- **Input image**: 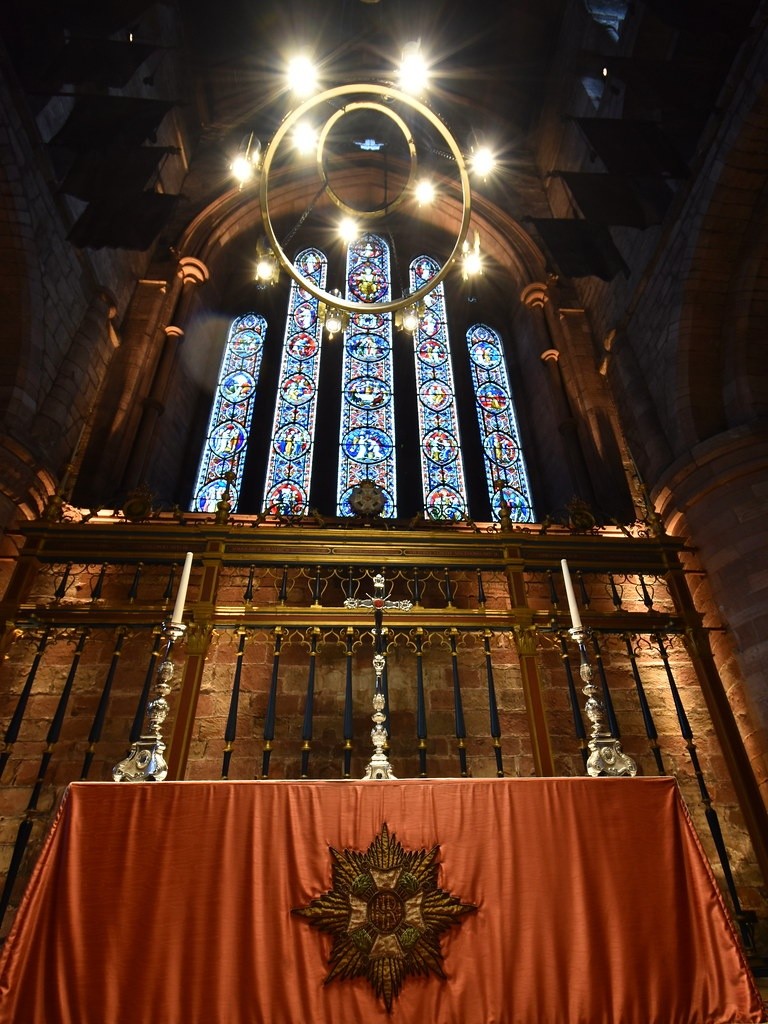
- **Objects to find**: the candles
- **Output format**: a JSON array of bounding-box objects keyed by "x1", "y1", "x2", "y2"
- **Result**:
[
  {"x1": 171, "y1": 552, "x2": 193, "y2": 623},
  {"x1": 561, "y1": 559, "x2": 582, "y2": 628}
]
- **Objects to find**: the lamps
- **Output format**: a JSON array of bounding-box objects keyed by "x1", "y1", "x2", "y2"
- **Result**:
[{"x1": 229, "y1": 78, "x2": 509, "y2": 341}]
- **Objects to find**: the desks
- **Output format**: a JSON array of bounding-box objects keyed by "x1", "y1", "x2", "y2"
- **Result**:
[{"x1": 68, "y1": 776, "x2": 678, "y2": 1024}]
